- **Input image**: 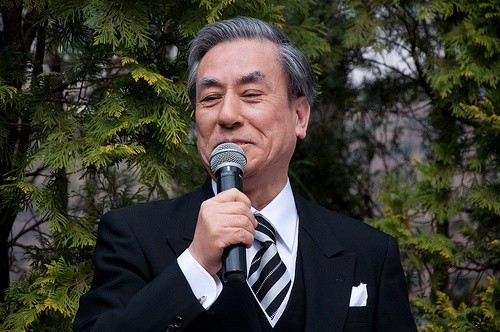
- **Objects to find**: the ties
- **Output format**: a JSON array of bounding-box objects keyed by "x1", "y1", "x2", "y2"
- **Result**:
[{"x1": 246, "y1": 213, "x2": 292, "y2": 320}]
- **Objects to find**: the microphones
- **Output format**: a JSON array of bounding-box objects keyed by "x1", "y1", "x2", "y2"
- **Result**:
[{"x1": 208, "y1": 141, "x2": 247, "y2": 284}]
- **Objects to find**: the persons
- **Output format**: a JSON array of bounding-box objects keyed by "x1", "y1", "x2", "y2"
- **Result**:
[{"x1": 72, "y1": 16, "x2": 414, "y2": 332}]
